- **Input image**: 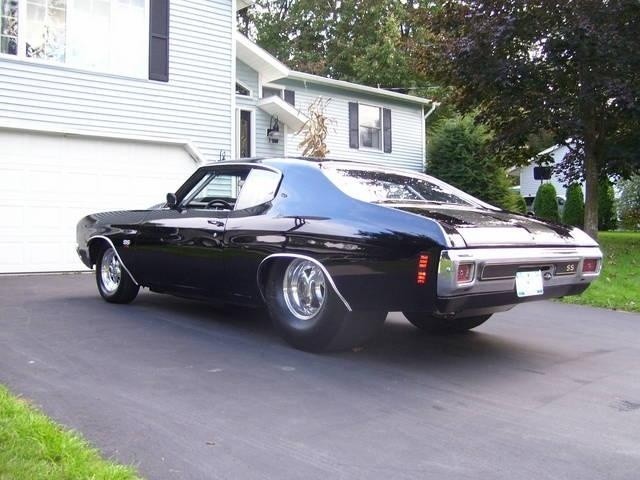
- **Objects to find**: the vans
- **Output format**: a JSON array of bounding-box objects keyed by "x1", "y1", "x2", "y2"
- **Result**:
[{"x1": 520, "y1": 193, "x2": 568, "y2": 220}]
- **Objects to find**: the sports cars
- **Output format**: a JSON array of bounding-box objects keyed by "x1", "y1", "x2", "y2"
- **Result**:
[{"x1": 76, "y1": 155, "x2": 604, "y2": 351}]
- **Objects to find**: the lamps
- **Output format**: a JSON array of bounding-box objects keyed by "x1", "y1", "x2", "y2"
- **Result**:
[{"x1": 267, "y1": 113, "x2": 283, "y2": 143}]
